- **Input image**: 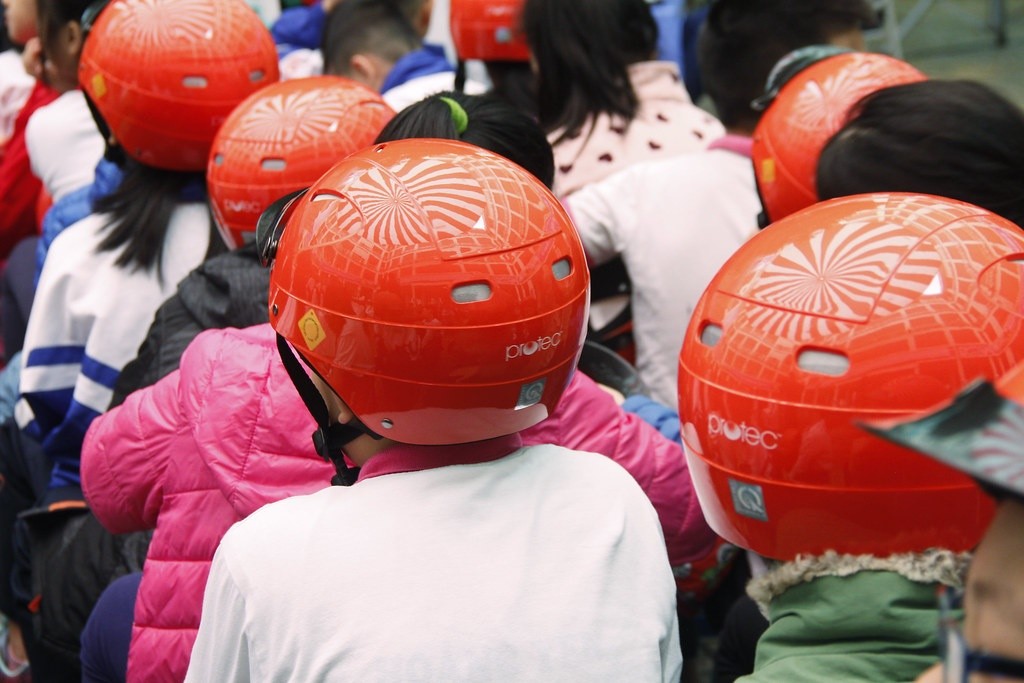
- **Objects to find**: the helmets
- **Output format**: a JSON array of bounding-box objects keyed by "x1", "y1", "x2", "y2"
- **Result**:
[
  {"x1": 268, "y1": 136, "x2": 591, "y2": 446},
  {"x1": 77, "y1": 0, "x2": 280, "y2": 170},
  {"x1": 450, "y1": 0, "x2": 532, "y2": 62},
  {"x1": 860, "y1": 359, "x2": 1024, "y2": 489},
  {"x1": 677, "y1": 190, "x2": 1024, "y2": 562},
  {"x1": 206, "y1": 76, "x2": 398, "y2": 252},
  {"x1": 751, "y1": 50, "x2": 929, "y2": 226}
]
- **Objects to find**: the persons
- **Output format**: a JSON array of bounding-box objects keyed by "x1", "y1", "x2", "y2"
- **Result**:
[
  {"x1": 678, "y1": 196, "x2": 1019, "y2": 683},
  {"x1": 1, "y1": 2, "x2": 1022, "y2": 680},
  {"x1": 176, "y1": 140, "x2": 688, "y2": 683}
]
